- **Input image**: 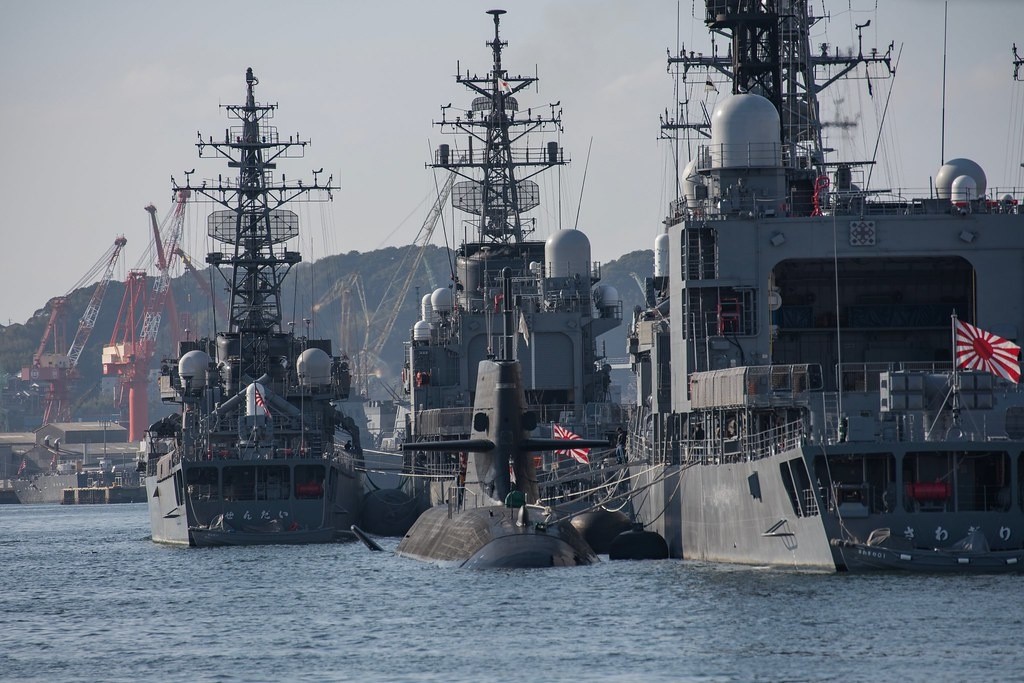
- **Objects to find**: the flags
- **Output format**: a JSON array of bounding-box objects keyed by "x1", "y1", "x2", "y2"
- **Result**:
[
  {"x1": 17, "y1": 459, "x2": 27, "y2": 475},
  {"x1": 508, "y1": 464, "x2": 516, "y2": 484},
  {"x1": 553, "y1": 423, "x2": 591, "y2": 464},
  {"x1": 498, "y1": 77, "x2": 513, "y2": 92},
  {"x1": 256, "y1": 386, "x2": 272, "y2": 419},
  {"x1": 954, "y1": 319, "x2": 1021, "y2": 385},
  {"x1": 518, "y1": 313, "x2": 529, "y2": 347}
]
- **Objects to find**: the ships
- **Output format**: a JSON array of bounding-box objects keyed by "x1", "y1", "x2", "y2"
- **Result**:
[
  {"x1": 142, "y1": 64, "x2": 367, "y2": 545},
  {"x1": 396, "y1": 9, "x2": 633, "y2": 523},
  {"x1": 623, "y1": 1, "x2": 1022, "y2": 572},
  {"x1": 9, "y1": 459, "x2": 148, "y2": 506}
]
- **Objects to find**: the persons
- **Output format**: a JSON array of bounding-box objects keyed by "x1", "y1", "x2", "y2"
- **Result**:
[
  {"x1": 562, "y1": 273, "x2": 584, "y2": 308},
  {"x1": 688, "y1": 421, "x2": 724, "y2": 464},
  {"x1": 839, "y1": 411, "x2": 849, "y2": 443},
  {"x1": 615, "y1": 427, "x2": 627, "y2": 464},
  {"x1": 344, "y1": 439, "x2": 353, "y2": 451},
  {"x1": 731, "y1": 177, "x2": 749, "y2": 200}
]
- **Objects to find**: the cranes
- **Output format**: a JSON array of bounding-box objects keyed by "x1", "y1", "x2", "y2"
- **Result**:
[
  {"x1": 308, "y1": 155, "x2": 464, "y2": 395},
  {"x1": 17, "y1": 187, "x2": 232, "y2": 444}
]
